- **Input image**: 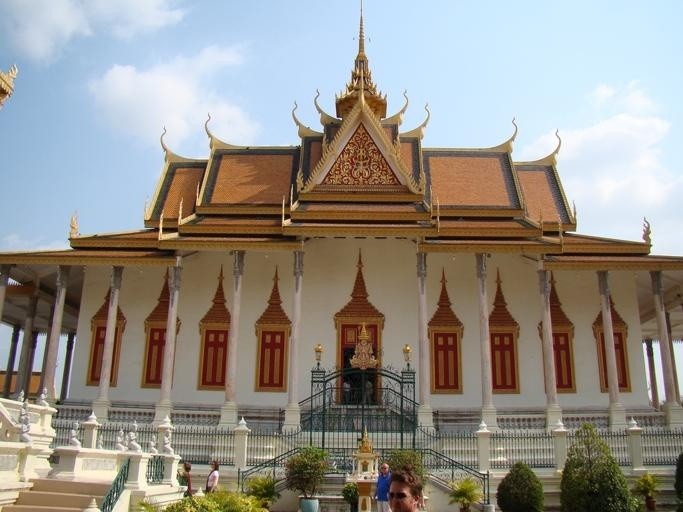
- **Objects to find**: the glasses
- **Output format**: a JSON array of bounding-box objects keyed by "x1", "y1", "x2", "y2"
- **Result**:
[{"x1": 387, "y1": 491, "x2": 415, "y2": 500}]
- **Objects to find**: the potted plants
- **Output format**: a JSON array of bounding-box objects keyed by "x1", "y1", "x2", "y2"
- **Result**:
[
  {"x1": 444, "y1": 474, "x2": 487, "y2": 512},
  {"x1": 283, "y1": 445, "x2": 340, "y2": 512},
  {"x1": 631, "y1": 470, "x2": 660, "y2": 512}
]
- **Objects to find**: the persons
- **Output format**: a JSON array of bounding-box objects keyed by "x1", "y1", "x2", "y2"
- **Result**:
[
  {"x1": 162, "y1": 429, "x2": 174, "y2": 455},
  {"x1": 343, "y1": 379, "x2": 350, "y2": 404},
  {"x1": 371, "y1": 463, "x2": 392, "y2": 512},
  {"x1": 387, "y1": 464, "x2": 422, "y2": 511},
  {"x1": 365, "y1": 379, "x2": 372, "y2": 405},
  {"x1": 19, "y1": 398, "x2": 29, "y2": 422},
  {"x1": 39, "y1": 387, "x2": 49, "y2": 406},
  {"x1": 206, "y1": 461, "x2": 218, "y2": 494},
  {"x1": 16, "y1": 415, "x2": 33, "y2": 442},
  {"x1": 178, "y1": 463, "x2": 192, "y2": 498},
  {"x1": 96, "y1": 435, "x2": 103, "y2": 448},
  {"x1": 126, "y1": 419, "x2": 141, "y2": 450},
  {"x1": 148, "y1": 435, "x2": 158, "y2": 454},
  {"x1": 16, "y1": 389, "x2": 24, "y2": 403},
  {"x1": 69, "y1": 423, "x2": 81, "y2": 447},
  {"x1": 115, "y1": 429, "x2": 126, "y2": 451}
]
